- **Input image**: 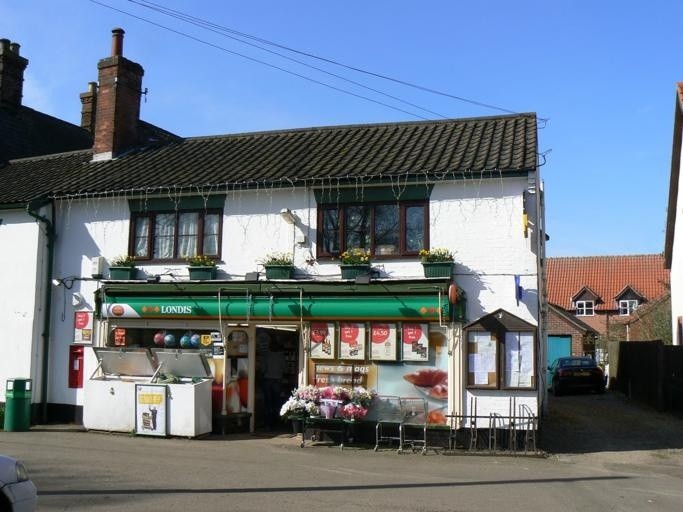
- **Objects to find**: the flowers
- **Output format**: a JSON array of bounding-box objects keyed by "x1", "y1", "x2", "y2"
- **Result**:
[
  {"x1": 263, "y1": 252, "x2": 294, "y2": 265},
  {"x1": 111, "y1": 255, "x2": 136, "y2": 267},
  {"x1": 417, "y1": 248, "x2": 458, "y2": 262},
  {"x1": 279, "y1": 383, "x2": 377, "y2": 421},
  {"x1": 181, "y1": 253, "x2": 216, "y2": 266},
  {"x1": 341, "y1": 247, "x2": 372, "y2": 264}
]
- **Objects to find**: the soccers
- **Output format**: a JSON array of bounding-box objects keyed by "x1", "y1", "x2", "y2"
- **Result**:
[{"x1": 155, "y1": 334, "x2": 201, "y2": 348}]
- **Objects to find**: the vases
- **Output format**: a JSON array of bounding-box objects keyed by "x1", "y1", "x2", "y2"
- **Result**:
[
  {"x1": 264, "y1": 264, "x2": 294, "y2": 279},
  {"x1": 340, "y1": 265, "x2": 370, "y2": 279},
  {"x1": 186, "y1": 265, "x2": 217, "y2": 280},
  {"x1": 108, "y1": 267, "x2": 133, "y2": 280},
  {"x1": 421, "y1": 261, "x2": 455, "y2": 277}
]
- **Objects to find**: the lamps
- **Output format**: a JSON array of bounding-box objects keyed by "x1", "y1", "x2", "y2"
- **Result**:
[
  {"x1": 51, "y1": 277, "x2": 95, "y2": 289},
  {"x1": 279, "y1": 208, "x2": 297, "y2": 224}
]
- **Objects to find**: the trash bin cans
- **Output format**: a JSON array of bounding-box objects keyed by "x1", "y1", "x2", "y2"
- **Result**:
[{"x1": 4, "y1": 378, "x2": 32, "y2": 432}]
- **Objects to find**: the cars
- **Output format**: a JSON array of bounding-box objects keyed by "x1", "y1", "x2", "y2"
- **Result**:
[
  {"x1": 0, "y1": 454, "x2": 38, "y2": 512},
  {"x1": 547, "y1": 356, "x2": 605, "y2": 396}
]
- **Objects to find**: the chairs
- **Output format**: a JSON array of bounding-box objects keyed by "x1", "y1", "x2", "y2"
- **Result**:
[{"x1": 373, "y1": 397, "x2": 428, "y2": 456}]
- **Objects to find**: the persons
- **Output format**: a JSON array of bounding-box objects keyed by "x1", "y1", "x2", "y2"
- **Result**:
[{"x1": 149, "y1": 406, "x2": 157, "y2": 430}]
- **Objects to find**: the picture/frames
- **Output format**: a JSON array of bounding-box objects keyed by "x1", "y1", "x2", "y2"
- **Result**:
[
  {"x1": 310, "y1": 321, "x2": 336, "y2": 361},
  {"x1": 400, "y1": 322, "x2": 429, "y2": 362},
  {"x1": 369, "y1": 321, "x2": 398, "y2": 362},
  {"x1": 73, "y1": 311, "x2": 94, "y2": 345},
  {"x1": 135, "y1": 384, "x2": 167, "y2": 438},
  {"x1": 337, "y1": 320, "x2": 366, "y2": 360}
]
